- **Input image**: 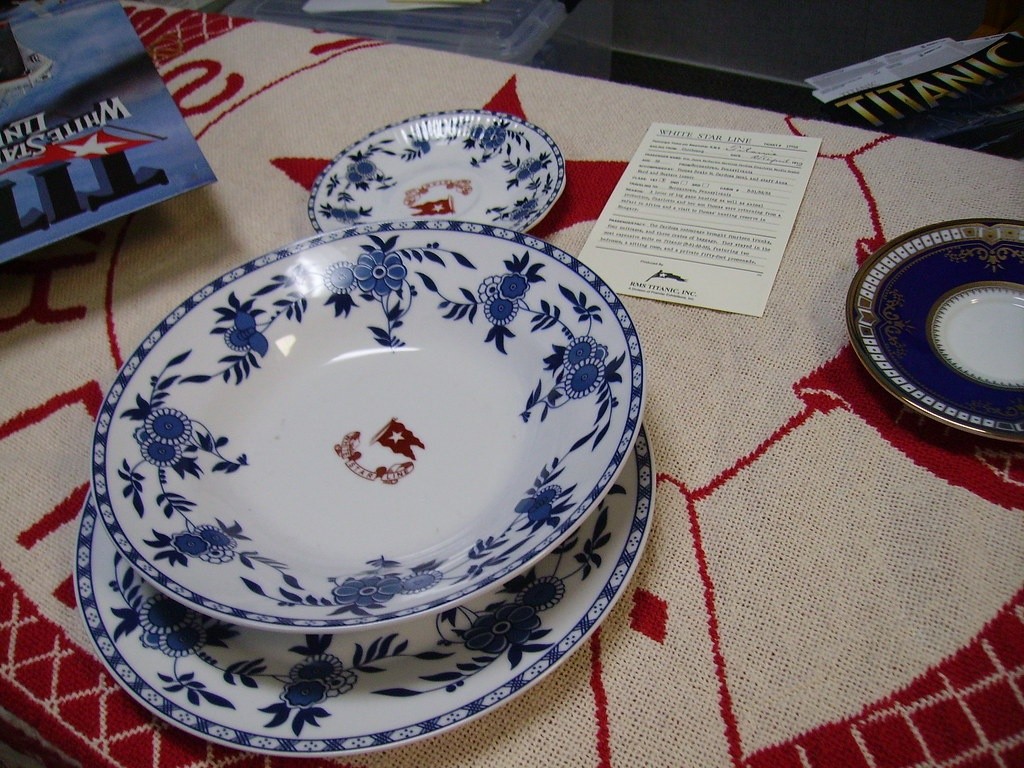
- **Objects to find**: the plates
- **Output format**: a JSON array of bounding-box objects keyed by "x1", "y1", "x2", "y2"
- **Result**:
[
  {"x1": 73, "y1": 421, "x2": 658, "y2": 757},
  {"x1": 308, "y1": 108, "x2": 567, "y2": 234},
  {"x1": 89, "y1": 220, "x2": 644, "y2": 633},
  {"x1": 844, "y1": 217, "x2": 1024, "y2": 445}
]
